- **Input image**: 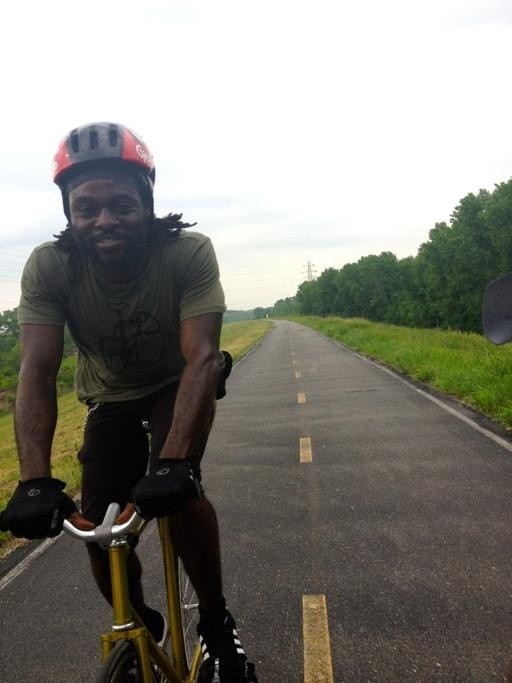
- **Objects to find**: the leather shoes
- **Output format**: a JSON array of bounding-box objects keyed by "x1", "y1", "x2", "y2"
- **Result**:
[
  {"x1": 127, "y1": 456, "x2": 196, "y2": 522},
  {"x1": 5, "y1": 477, "x2": 79, "y2": 541}
]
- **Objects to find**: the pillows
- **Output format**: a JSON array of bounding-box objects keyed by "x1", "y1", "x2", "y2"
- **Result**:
[{"x1": 50, "y1": 121, "x2": 157, "y2": 180}]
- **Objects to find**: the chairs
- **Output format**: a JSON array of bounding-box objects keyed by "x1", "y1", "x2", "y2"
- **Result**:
[{"x1": 196, "y1": 609, "x2": 245, "y2": 662}]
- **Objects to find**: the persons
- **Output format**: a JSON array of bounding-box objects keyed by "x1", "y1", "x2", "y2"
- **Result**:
[{"x1": 1, "y1": 120, "x2": 251, "y2": 683}]
